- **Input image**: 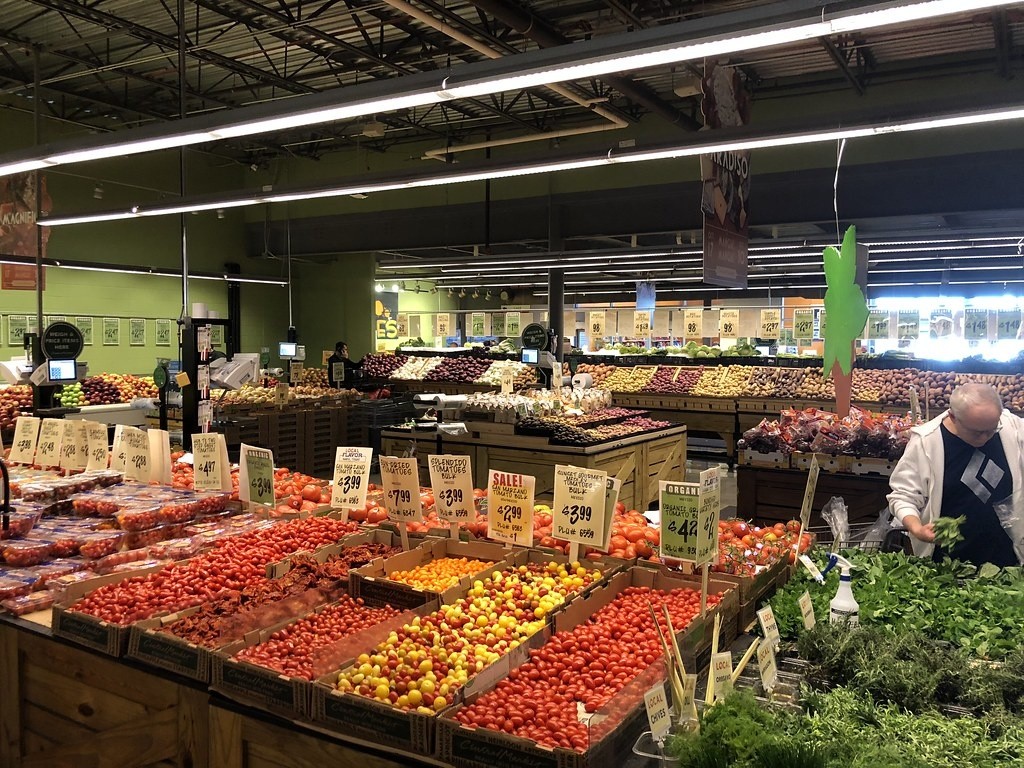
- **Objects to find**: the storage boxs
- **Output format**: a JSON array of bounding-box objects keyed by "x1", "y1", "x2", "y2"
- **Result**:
[
  {"x1": 54, "y1": 485, "x2": 817, "y2": 768},
  {"x1": 738, "y1": 448, "x2": 900, "y2": 476},
  {"x1": 391, "y1": 357, "x2": 1024, "y2": 421},
  {"x1": 145, "y1": 396, "x2": 365, "y2": 419}
]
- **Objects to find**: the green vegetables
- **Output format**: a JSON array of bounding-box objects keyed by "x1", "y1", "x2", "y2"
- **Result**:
[
  {"x1": 490, "y1": 337, "x2": 516, "y2": 354},
  {"x1": 653, "y1": 514, "x2": 1024, "y2": 768},
  {"x1": 394, "y1": 336, "x2": 424, "y2": 351}
]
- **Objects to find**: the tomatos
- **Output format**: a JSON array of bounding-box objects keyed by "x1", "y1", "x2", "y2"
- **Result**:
[{"x1": 0, "y1": 440, "x2": 812, "y2": 753}]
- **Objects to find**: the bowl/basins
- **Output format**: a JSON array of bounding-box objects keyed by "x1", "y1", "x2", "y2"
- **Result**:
[{"x1": 1, "y1": 465, "x2": 274, "y2": 615}]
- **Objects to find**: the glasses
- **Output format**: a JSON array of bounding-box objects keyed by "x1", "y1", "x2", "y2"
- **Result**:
[{"x1": 955, "y1": 418, "x2": 1003, "y2": 438}]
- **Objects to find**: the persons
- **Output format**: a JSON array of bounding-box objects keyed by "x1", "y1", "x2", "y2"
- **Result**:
[
  {"x1": 328, "y1": 342, "x2": 367, "y2": 390},
  {"x1": 886, "y1": 383, "x2": 1024, "y2": 568}
]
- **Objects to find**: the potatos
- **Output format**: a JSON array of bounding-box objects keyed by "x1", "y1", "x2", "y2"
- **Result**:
[{"x1": 741, "y1": 368, "x2": 1024, "y2": 413}]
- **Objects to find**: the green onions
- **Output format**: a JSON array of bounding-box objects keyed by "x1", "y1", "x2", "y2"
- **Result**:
[{"x1": 880, "y1": 349, "x2": 915, "y2": 361}]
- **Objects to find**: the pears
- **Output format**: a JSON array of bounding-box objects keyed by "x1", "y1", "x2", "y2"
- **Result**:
[
  {"x1": 512, "y1": 363, "x2": 615, "y2": 388},
  {"x1": 208, "y1": 385, "x2": 278, "y2": 404},
  {"x1": 690, "y1": 364, "x2": 753, "y2": 397},
  {"x1": 598, "y1": 367, "x2": 654, "y2": 392}
]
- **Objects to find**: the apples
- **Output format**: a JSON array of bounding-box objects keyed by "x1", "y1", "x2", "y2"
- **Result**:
[
  {"x1": 359, "y1": 352, "x2": 494, "y2": 384},
  {"x1": 640, "y1": 364, "x2": 704, "y2": 394},
  {"x1": 288, "y1": 368, "x2": 363, "y2": 396},
  {"x1": 55, "y1": 372, "x2": 160, "y2": 407},
  {"x1": 0, "y1": 384, "x2": 39, "y2": 429}
]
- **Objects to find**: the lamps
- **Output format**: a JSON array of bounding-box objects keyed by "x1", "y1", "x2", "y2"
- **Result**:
[
  {"x1": 36, "y1": 103, "x2": 1023, "y2": 226},
  {"x1": 0, "y1": 0, "x2": 1024, "y2": 177},
  {"x1": 0, "y1": 220, "x2": 289, "y2": 285},
  {"x1": 379, "y1": 225, "x2": 1024, "y2": 300}
]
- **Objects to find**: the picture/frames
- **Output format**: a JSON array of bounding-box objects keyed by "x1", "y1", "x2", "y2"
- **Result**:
[
  {"x1": 47, "y1": 316, "x2": 66, "y2": 326},
  {"x1": 102, "y1": 318, "x2": 120, "y2": 345},
  {"x1": 7, "y1": 315, "x2": 48, "y2": 345},
  {"x1": 0, "y1": 315, "x2": 3, "y2": 344},
  {"x1": 75, "y1": 317, "x2": 94, "y2": 345},
  {"x1": 155, "y1": 319, "x2": 172, "y2": 345},
  {"x1": 129, "y1": 319, "x2": 146, "y2": 345}
]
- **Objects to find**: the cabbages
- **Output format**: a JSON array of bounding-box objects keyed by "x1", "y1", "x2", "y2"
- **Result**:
[{"x1": 603, "y1": 342, "x2": 762, "y2": 358}]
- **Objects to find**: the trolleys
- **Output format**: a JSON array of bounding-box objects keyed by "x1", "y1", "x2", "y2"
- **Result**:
[{"x1": 801, "y1": 521, "x2": 908, "y2": 558}]
